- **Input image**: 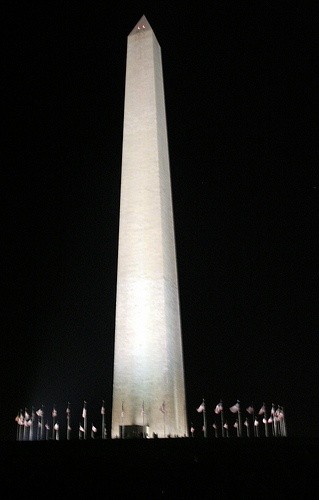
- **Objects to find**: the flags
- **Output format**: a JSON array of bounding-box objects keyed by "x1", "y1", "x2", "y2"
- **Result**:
[{"x1": 14, "y1": 397, "x2": 287, "y2": 436}]
